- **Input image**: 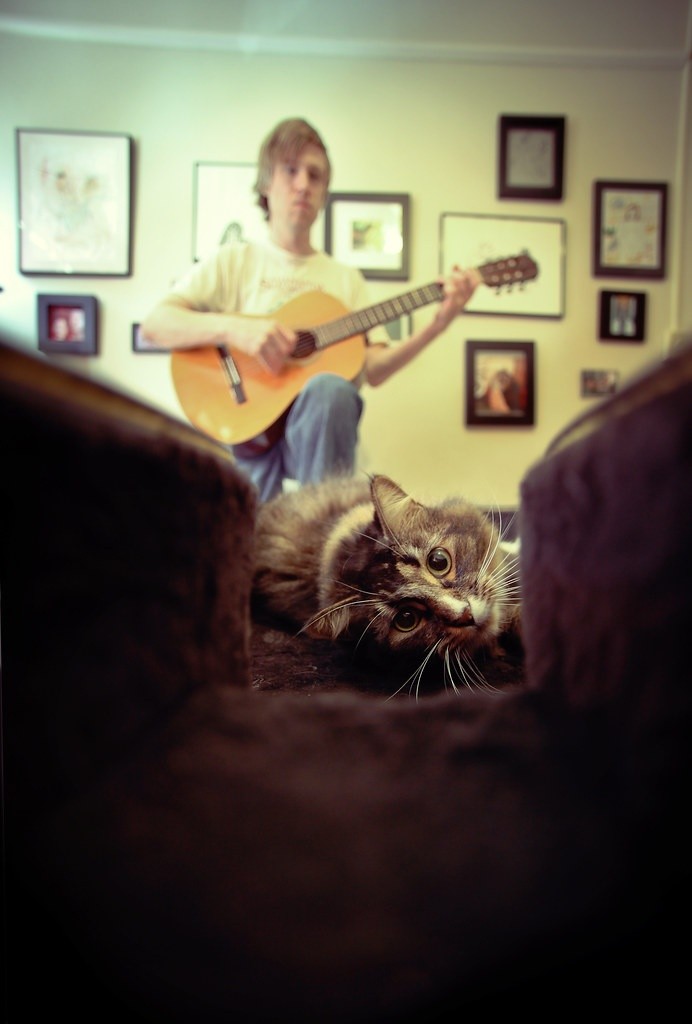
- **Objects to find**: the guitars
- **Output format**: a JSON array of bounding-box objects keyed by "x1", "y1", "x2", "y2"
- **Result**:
[{"x1": 170, "y1": 248, "x2": 540, "y2": 460}]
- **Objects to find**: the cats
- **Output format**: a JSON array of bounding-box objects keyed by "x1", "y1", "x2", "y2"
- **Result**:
[{"x1": 252, "y1": 475, "x2": 523, "y2": 704}]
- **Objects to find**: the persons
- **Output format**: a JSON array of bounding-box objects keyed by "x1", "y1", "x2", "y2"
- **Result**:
[{"x1": 138, "y1": 117, "x2": 480, "y2": 508}]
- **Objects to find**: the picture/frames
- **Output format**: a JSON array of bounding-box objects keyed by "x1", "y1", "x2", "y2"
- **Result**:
[
  {"x1": 15, "y1": 128, "x2": 130, "y2": 277},
  {"x1": 382, "y1": 314, "x2": 410, "y2": 350},
  {"x1": 326, "y1": 192, "x2": 409, "y2": 283},
  {"x1": 597, "y1": 289, "x2": 645, "y2": 342},
  {"x1": 438, "y1": 213, "x2": 565, "y2": 317},
  {"x1": 465, "y1": 340, "x2": 534, "y2": 425},
  {"x1": 497, "y1": 114, "x2": 566, "y2": 200},
  {"x1": 38, "y1": 293, "x2": 97, "y2": 353},
  {"x1": 193, "y1": 161, "x2": 262, "y2": 264},
  {"x1": 590, "y1": 178, "x2": 667, "y2": 279}
]
